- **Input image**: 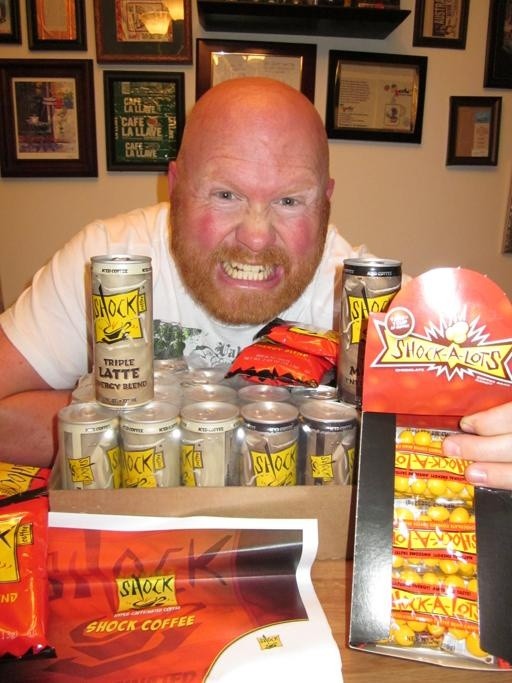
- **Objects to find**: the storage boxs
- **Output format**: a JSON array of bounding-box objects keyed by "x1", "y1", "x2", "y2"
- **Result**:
[{"x1": 47, "y1": 452, "x2": 356, "y2": 561}]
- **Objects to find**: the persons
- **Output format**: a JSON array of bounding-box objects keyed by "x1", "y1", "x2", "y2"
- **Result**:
[{"x1": 0, "y1": 76, "x2": 512, "y2": 492}]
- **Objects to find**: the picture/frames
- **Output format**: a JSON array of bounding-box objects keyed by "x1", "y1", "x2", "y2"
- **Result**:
[
  {"x1": 483, "y1": 1, "x2": 512, "y2": 91},
  {"x1": 196, "y1": 36, "x2": 317, "y2": 110},
  {"x1": 410, "y1": 0, "x2": 470, "y2": 51},
  {"x1": 0, "y1": 57, "x2": 99, "y2": 181},
  {"x1": 323, "y1": 49, "x2": 428, "y2": 146},
  {"x1": 444, "y1": 94, "x2": 503, "y2": 168},
  {"x1": 92, "y1": 1, "x2": 193, "y2": 67},
  {"x1": 29, "y1": 0, "x2": 89, "y2": 53},
  {"x1": 102, "y1": 69, "x2": 187, "y2": 173},
  {"x1": 1, "y1": 1, "x2": 22, "y2": 47}
]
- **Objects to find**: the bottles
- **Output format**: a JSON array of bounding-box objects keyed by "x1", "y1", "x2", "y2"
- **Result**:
[
  {"x1": 59, "y1": 355, "x2": 239, "y2": 404},
  {"x1": 300, "y1": 399, "x2": 358, "y2": 483},
  {"x1": 91, "y1": 254, "x2": 154, "y2": 408},
  {"x1": 181, "y1": 401, "x2": 238, "y2": 486},
  {"x1": 339, "y1": 258, "x2": 400, "y2": 405},
  {"x1": 58, "y1": 409, "x2": 117, "y2": 488},
  {"x1": 294, "y1": 383, "x2": 336, "y2": 406},
  {"x1": 239, "y1": 403, "x2": 297, "y2": 488},
  {"x1": 119, "y1": 407, "x2": 181, "y2": 484},
  {"x1": 238, "y1": 385, "x2": 290, "y2": 401}
]
